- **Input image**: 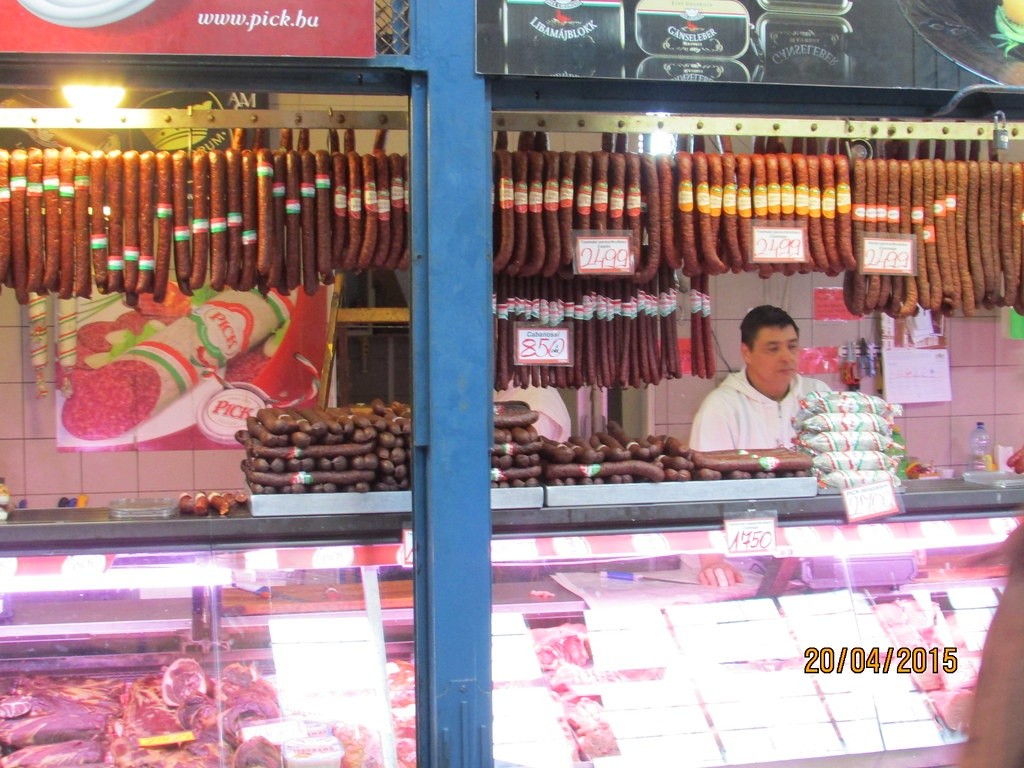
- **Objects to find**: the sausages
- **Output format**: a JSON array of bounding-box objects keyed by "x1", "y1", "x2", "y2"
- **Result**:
[
  {"x1": 1, "y1": 121, "x2": 1024, "y2": 391},
  {"x1": 177, "y1": 407, "x2": 814, "y2": 517}
]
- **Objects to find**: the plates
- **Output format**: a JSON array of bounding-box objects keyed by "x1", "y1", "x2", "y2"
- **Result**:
[
  {"x1": 919, "y1": 477, "x2": 940, "y2": 480},
  {"x1": 108, "y1": 497, "x2": 179, "y2": 520}
]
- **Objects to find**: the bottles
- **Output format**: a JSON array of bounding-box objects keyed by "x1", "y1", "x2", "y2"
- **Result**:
[
  {"x1": 967, "y1": 422, "x2": 989, "y2": 471},
  {"x1": 0, "y1": 478, "x2": 9, "y2": 521}
]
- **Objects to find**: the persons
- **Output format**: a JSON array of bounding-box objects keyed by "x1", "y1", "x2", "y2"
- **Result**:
[
  {"x1": 688, "y1": 306, "x2": 833, "y2": 452},
  {"x1": 960, "y1": 445, "x2": 1024, "y2": 768}
]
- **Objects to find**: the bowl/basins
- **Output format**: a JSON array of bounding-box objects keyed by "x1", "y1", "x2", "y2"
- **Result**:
[{"x1": 937, "y1": 469, "x2": 955, "y2": 479}]
